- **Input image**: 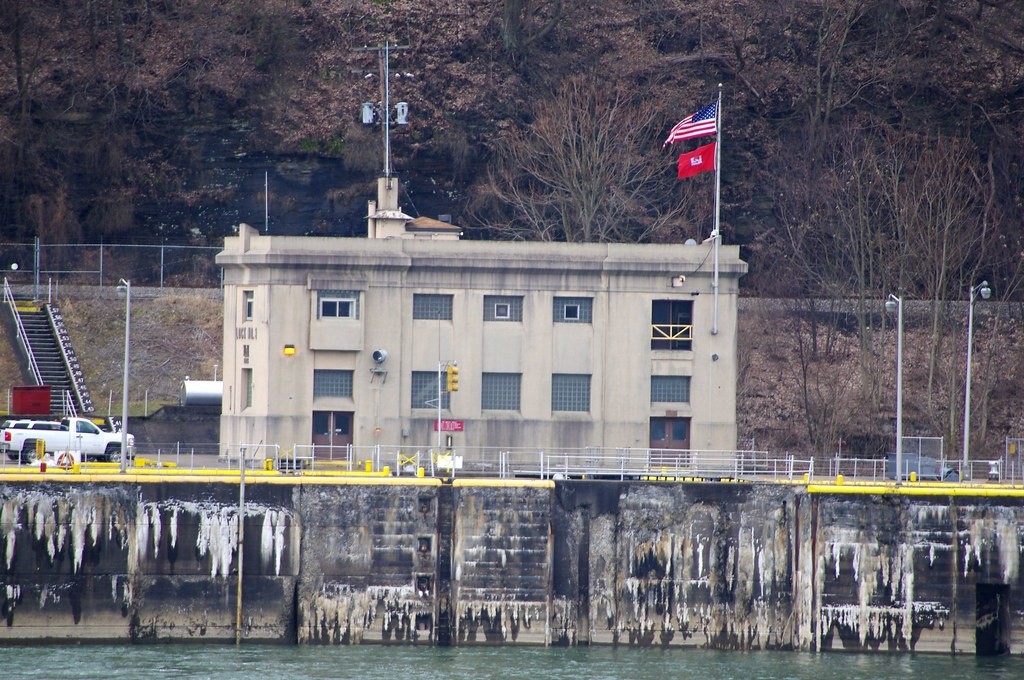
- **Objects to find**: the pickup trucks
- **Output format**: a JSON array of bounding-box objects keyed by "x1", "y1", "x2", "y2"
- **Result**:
[{"x1": 0, "y1": 417, "x2": 138, "y2": 464}]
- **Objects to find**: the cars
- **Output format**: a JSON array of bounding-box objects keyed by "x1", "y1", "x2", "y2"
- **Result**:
[{"x1": 2, "y1": 419, "x2": 68, "y2": 430}]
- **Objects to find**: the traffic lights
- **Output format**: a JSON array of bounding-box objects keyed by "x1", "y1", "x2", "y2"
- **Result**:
[{"x1": 447, "y1": 365, "x2": 459, "y2": 393}]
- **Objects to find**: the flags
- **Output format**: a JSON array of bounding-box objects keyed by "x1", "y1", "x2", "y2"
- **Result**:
[
  {"x1": 663, "y1": 99, "x2": 718, "y2": 148},
  {"x1": 678, "y1": 142, "x2": 717, "y2": 178}
]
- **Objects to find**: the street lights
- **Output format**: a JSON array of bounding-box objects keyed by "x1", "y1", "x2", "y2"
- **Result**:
[
  {"x1": 885, "y1": 292, "x2": 903, "y2": 487},
  {"x1": 963, "y1": 281, "x2": 990, "y2": 480},
  {"x1": 116, "y1": 278, "x2": 131, "y2": 473}
]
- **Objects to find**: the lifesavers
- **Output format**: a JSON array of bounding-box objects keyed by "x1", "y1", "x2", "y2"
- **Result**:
[{"x1": 58, "y1": 454, "x2": 75, "y2": 469}]
